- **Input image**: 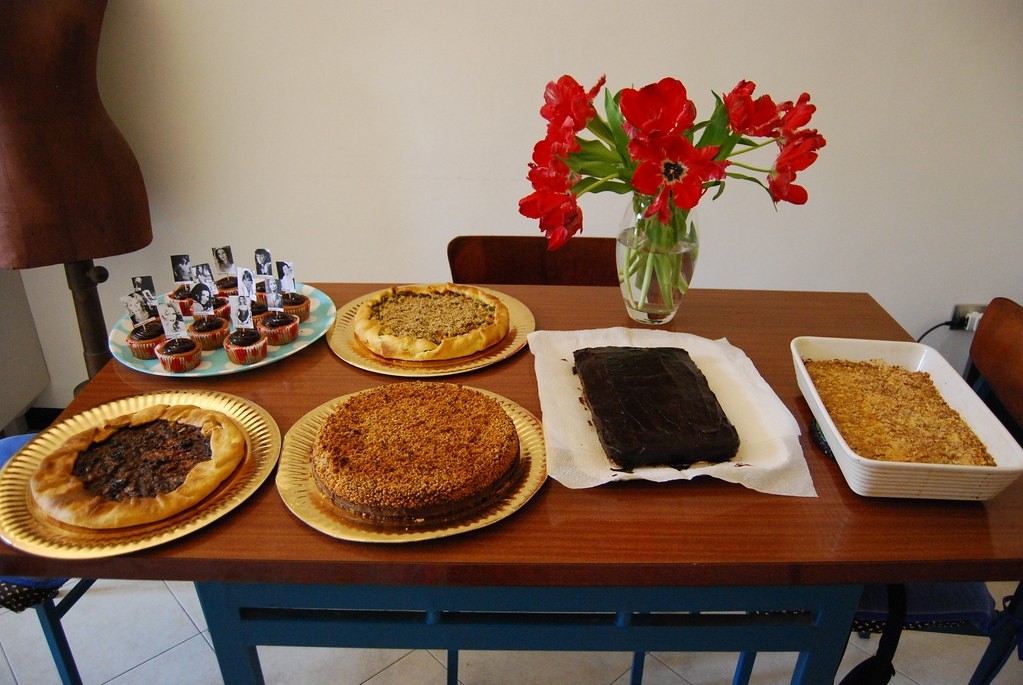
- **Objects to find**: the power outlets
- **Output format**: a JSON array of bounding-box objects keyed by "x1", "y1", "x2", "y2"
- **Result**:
[{"x1": 947, "y1": 301, "x2": 988, "y2": 331}]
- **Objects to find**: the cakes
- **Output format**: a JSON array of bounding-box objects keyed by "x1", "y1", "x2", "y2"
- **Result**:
[
  {"x1": 313, "y1": 382, "x2": 521, "y2": 525},
  {"x1": 572, "y1": 346, "x2": 742, "y2": 469}
]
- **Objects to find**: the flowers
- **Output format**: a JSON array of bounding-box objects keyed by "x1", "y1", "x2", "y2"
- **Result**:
[{"x1": 519, "y1": 74, "x2": 825, "y2": 249}]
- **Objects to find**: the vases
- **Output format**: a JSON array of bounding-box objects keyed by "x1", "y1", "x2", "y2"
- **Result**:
[{"x1": 611, "y1": 192, "x2": 697, "y2": 326}]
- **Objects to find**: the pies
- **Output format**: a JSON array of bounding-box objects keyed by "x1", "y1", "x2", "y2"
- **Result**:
[
  {"x1": 29, "y1": 404, "x2": 245, "y2": 528},
  {"x1": 356, "y1": 281, "x2": 510, "y2": 361}
]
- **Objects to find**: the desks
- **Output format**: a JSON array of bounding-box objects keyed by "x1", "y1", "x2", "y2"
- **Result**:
[{"x1": 0, "y1": 282, "x2": 1023, "y2": 685}]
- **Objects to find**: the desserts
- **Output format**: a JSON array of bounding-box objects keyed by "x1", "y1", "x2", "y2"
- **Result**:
[{"x1": 125, "y1": 276, "x2": 311, "y2": 373}]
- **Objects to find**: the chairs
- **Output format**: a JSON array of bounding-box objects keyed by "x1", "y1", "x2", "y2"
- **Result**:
[
  {"x1": 731, "y1": 297, "x2": 1023, "y2": 685},
  {"x1": 0, "y1": 434, "x2": 114, "y2": 685},
  {"x1": 444, "y1": 234, "x2": 645, "y2": 685}
]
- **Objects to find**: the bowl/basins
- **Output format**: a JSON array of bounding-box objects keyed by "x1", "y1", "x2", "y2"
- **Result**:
[{"x1": 790, "y1": 337, "x2": 1023, "y2": 500}]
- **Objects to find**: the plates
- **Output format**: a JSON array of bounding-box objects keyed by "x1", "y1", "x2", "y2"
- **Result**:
[
  {"x1": 276, "y1": 382, "x2": 554, "y2": 542},
  {"x1": 325, "y1": 283, "x2": 534, "y2": 376},
  {"x1": 108, "y1": 280, "x2": 335, "y2": 376},
  {"x1": 0, "y1": 390, "x2": 282, "y2": 558}
]
